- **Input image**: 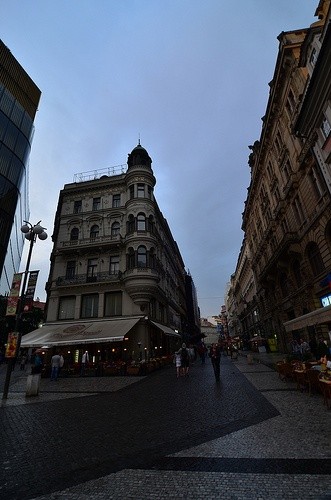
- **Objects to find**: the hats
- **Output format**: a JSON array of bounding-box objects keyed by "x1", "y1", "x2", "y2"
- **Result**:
[{"x1": 182, "y1": 342, "x2": 186, "y2": 349}]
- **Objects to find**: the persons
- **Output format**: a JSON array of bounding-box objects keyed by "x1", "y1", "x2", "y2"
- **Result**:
[
  {"x1": 19, "y1": 356, "x2": 26, "y2": 371},
  {"x1": 298, "y1": 337, "x2": 312, "y2": 361},
  {"x1": 81, "y1": 350, "x2": 89, "y2": 376},
  {"x1": 171, "y1": 343, "x2": 195, "y2": 377},
  {"x1": 50, "y1": 351, "x2": 64, "y2": 382},
  {"x1": 199, "y1": 343, "x2": 207, "y2": 365},
  {"x1": 209, "y1": 343, "x2": 221, "y2": 382}
]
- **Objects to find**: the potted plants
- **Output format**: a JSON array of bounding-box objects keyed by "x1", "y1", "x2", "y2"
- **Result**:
[{"x1": 126, "y1": 363, "x2": 142, "y2": 375}]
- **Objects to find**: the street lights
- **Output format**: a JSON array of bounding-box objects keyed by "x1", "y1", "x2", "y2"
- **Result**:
[{"x1": 3, "y1": 218, "x2": 48, "y2": 400}]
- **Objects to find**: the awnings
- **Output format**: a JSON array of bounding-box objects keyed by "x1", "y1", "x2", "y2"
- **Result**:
[
  {"x1": 3, "y1": 313, "x2": 145, "y2": 347},
  {"x1": 150, "y1": 321, "x2": 182, "y2": 339},
  {"x1": 283, "y1": 304, "x2": 331, "y2": 332}
]
